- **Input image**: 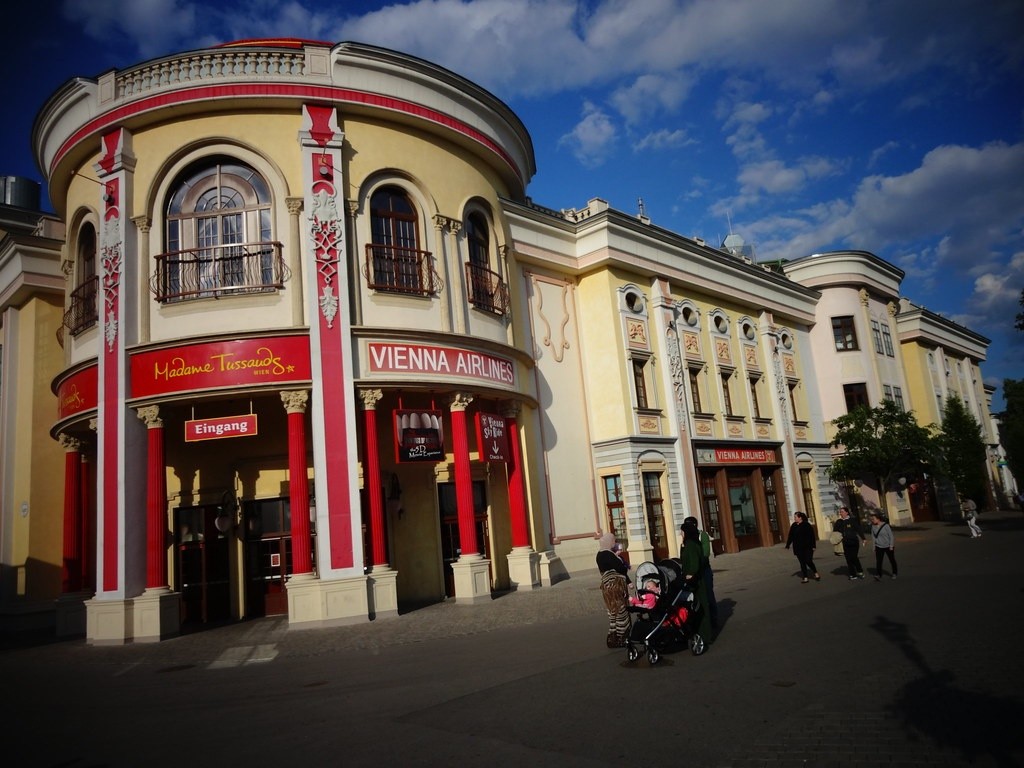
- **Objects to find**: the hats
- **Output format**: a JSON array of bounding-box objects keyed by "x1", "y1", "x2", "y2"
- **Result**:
[{"x1": 615, "y1": 543, "x2": 623, "y2": 550}]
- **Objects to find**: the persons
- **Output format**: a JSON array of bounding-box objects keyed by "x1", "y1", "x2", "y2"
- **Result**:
[
  {"x1": 597, "y1": 533, "x2": 631, "y2": 646},
  {"x1": 833, "y1": 507, "x2": 866, "y2": 580},
  {"x1": 625, "y1": 582, "x2": 660, "y2": 609},
  {"x1": 685, "y1": 517, "x2": 717, "y2": 617},
  {"x1": 666, "y1": 522, "x2": 711, "y2": 644},
  {"x1": 785, "y1": 512, "x2": 820, "y2": 583},
  {"x1": 868, "y1": 514, "x2": 897, "y2": 581},
  {"x1": 961, "y1": 496, "x2": 983, "y2": 538}
]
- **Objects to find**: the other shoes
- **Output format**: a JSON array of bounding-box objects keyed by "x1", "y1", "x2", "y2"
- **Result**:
[
  {"x1": 874, "y1": 575, "x2": 881, "y2": 582},
  {"x1": 607, "y1": 633, "x2": 618, "y2": 647},
  {"x1": 971, "y1": 536, "x2": 975, "y2": 539},
  {"x1": 815, "y1": 573, "x2": 820, "y2": 580},
  {"x1": 700, "y1": 640, "x2": 713, "y2": 646},
  {"x1": 978, "y1": 533, "x2": 984, "y2": 537},
  {"x1": 619, "y1": 635, "x2": 629, "y2": 647},
  {"x1": 801, "y1": 580, "x2": 809, "y2": 584},
  {"x1": 849, "y1": 576, "x2": 857, "y2": 580},
  {"x1": 891, "y1": 574, "x2": 897, "y2": 580},
  {"x1": 859, "y1": 573, "x2": 865, "y2": 579}
]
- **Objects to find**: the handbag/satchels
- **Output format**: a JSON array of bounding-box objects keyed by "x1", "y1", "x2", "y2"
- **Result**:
[{"x1": 965, "y1": 511, "x2": 973, "y2": 520}]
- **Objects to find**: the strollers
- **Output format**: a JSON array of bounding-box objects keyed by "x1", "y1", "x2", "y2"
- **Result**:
[{"x1": 623, "y1": 557, "x2": 706, "y2": 666}]
